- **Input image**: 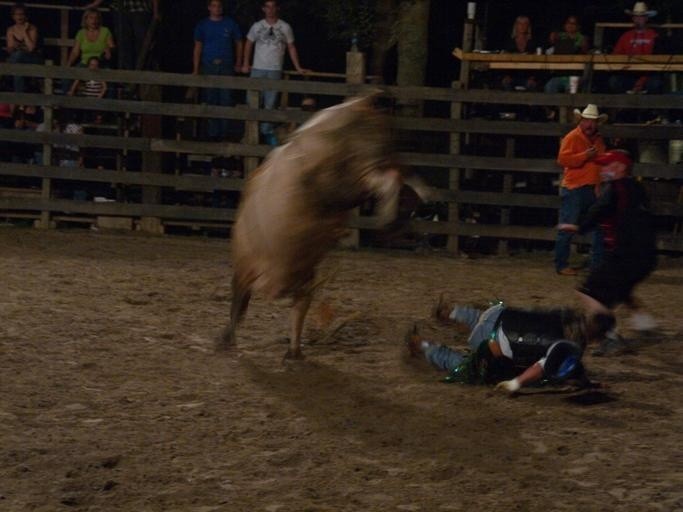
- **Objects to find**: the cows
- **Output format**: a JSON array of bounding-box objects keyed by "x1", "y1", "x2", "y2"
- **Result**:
[{"x1": 214, "y1": 86, "x2": 448, "y2": 361}]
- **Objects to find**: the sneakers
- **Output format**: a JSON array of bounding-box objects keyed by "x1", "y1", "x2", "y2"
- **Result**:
[{"x1": 408, "y1": 302, "x2": 457, "y2": 363}]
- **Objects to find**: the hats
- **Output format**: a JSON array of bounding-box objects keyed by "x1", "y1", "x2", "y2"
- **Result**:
[
  {"x1": 624, "y1": 2, "x2": 657, "y2": 19},
  {"x1": 592, "y1": 151, "x2": 633, "y2": 167},
  {"x1": 573, "y1": 102, "x2": 609, "y2": 124}
]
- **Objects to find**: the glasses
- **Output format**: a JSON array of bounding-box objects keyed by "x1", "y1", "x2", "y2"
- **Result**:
[{"x1": 269, "y1": 26, "x2": 273, "y2": 36}]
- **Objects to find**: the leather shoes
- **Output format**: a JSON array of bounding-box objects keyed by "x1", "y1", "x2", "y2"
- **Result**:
[{"x1": 558, "y1": 267, "x2": 579, "y2": 277}]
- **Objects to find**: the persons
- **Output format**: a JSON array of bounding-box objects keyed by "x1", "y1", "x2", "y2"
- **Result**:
[
  {"x1": 552, "y1": 103, "x2": 608, "y2": 276},
  {"x1": 554, "y1": 147, "x2": 659, "y2": 358},
  {"x1": 611, "y1": 1, "x2": 661, "y2": 94},
  {"x1": 404, "y1": 294, "x2": 616, "y2": 397},
  {"x1": 497, "y1": 15, "x2": 589, "y2": 119}
]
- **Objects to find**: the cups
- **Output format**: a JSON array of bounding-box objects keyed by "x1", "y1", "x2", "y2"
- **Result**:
[{"x1": 569, "y1": 75, "x2": 580, "y2": 94}]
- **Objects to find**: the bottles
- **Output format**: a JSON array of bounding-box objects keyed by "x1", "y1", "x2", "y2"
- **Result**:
[{"x1": 349, "y1": 31, "x2": 359, "y2": 51}]
